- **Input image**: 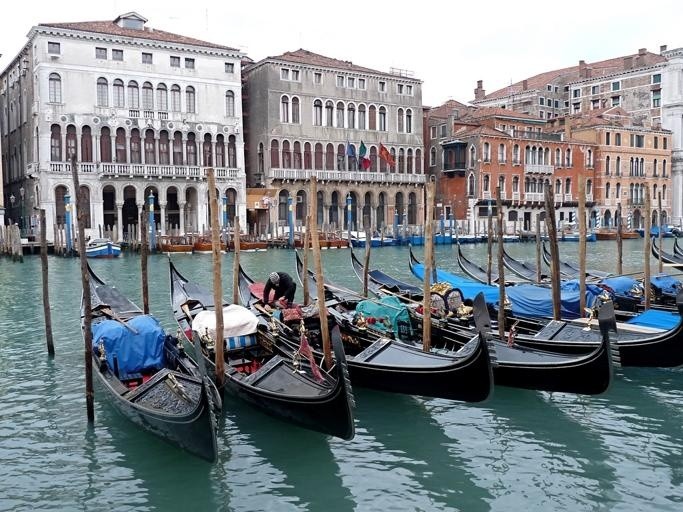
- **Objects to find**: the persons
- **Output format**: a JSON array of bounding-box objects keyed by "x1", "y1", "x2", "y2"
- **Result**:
[{"x1": 261, "y1": 272, "x2": 296, "y2": 311}]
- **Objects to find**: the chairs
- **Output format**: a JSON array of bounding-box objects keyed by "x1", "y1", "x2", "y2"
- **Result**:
[
  {"x1": 223, "y1": 340, "x2": 253, "y2": 374},
  {"x1": 112, "y1": 354, "x2": 143, "y2": 389}
]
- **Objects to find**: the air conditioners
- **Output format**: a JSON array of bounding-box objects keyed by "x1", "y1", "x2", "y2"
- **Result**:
[
  {"x1": 279, "y1": 196, "x2": 286, "y2": 203},
  {"x1": 297, "y1": 196, "x2": 302, "y2": 203}
]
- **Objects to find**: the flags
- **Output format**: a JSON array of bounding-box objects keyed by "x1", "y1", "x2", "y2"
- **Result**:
[
  {"x1": 377, "y1": 143, "x2": 396, "y2": 168},
  {"x1": 359, "y1": 141, "x2": 370, "y2": 169},
  {"x1": 346, "y1": 141, "x2": 358, "y2": 164}
]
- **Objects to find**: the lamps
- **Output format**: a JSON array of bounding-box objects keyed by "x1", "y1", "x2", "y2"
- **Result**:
[{"x1": 10, "y1": 194, "x2": 21, "y2": 208}]
